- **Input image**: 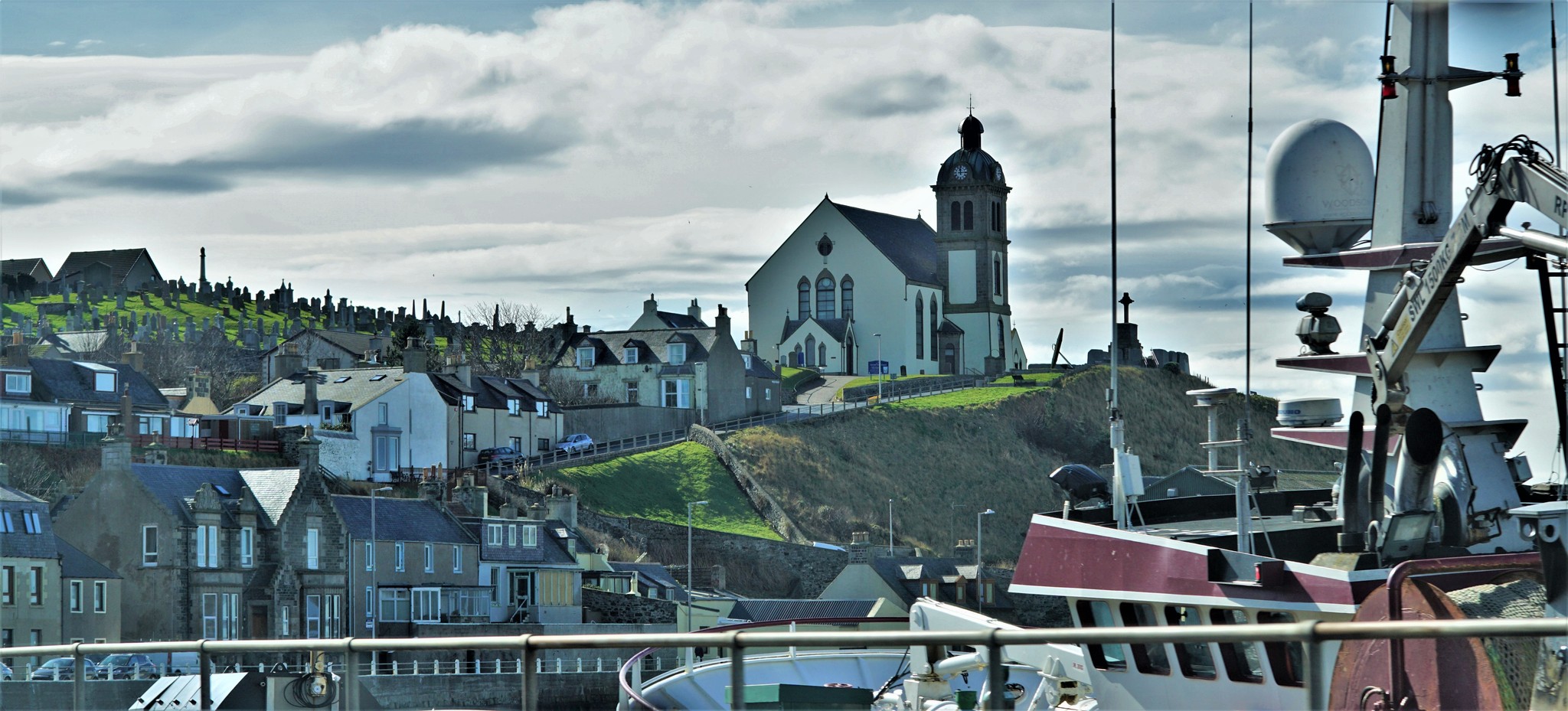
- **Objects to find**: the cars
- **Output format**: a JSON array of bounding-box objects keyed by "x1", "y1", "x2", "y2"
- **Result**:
[
  {"x1": 30, "y1": 657, "x2": 99, "y2": 680},
  {"x1": 553, "y1": 433, "x2": 594, "y2": 456},
  {"x1": 1, "y1": 663, "x2": 15, "y2": 681}
]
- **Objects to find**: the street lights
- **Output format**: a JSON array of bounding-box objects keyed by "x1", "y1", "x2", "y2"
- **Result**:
[
  {"x1": 688, "y1": 501, "x2": 708, "y2": 632},
  {"x1": 889, "y1": 499, "x2": 894, "y2": 557},
  {"x1": 873, "y1": 334, "x2": 881, "y2": 403},
  {"x1": 371, "y1": 487, "x2": 395, "y2": 673},
  {"x1": 978, "y1": 510, "x2": 994, "y2": 614}
]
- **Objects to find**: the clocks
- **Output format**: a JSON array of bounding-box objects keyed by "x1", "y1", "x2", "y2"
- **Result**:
[
  {"x1": 996, "y1": 166, "x2": 1002, "y2": 181},
  {"x1": 953, "y1": 165, "x2": 968, "y2": 180}
]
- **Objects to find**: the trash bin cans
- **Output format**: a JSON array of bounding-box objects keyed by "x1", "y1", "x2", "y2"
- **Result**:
[
  {"x1": 900, "y1": 366, "x2": 907, "y2": 375},
  {"x1": 920, "y1": 370, "x2": 924, "y2": 375},
  {"x1": 583, "y1": 607, "x2": 603, "y2": 624}
]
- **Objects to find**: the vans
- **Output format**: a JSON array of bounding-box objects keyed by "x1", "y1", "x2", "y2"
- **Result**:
[
  {"x1": 145, "y1": 653, "x2": 214, "y2": 677},
  {"x1": 813, "y1": 542, "x2": 846, "y2": 551}
]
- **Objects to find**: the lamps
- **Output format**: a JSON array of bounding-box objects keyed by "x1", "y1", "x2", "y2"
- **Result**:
[
  {"x1": 1378, "y1": 510, "x2": 1435, "y2": 567},
  {"x1": 1049, "y1": 464, "x2": 1110, "y2": 506}
]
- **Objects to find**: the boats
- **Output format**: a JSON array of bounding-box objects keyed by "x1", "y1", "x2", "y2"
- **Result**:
[{"x1": 610, "y1": 1, "x2": 1568, "y2": 709}]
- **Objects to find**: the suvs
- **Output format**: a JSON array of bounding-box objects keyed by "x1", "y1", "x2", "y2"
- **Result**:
[
  {"x1": 96, "y1": 653, "x2": 157, "y2": 679},
  {"x1": 477, "y1": 447, "x2": 524, "y2": 469}
]
